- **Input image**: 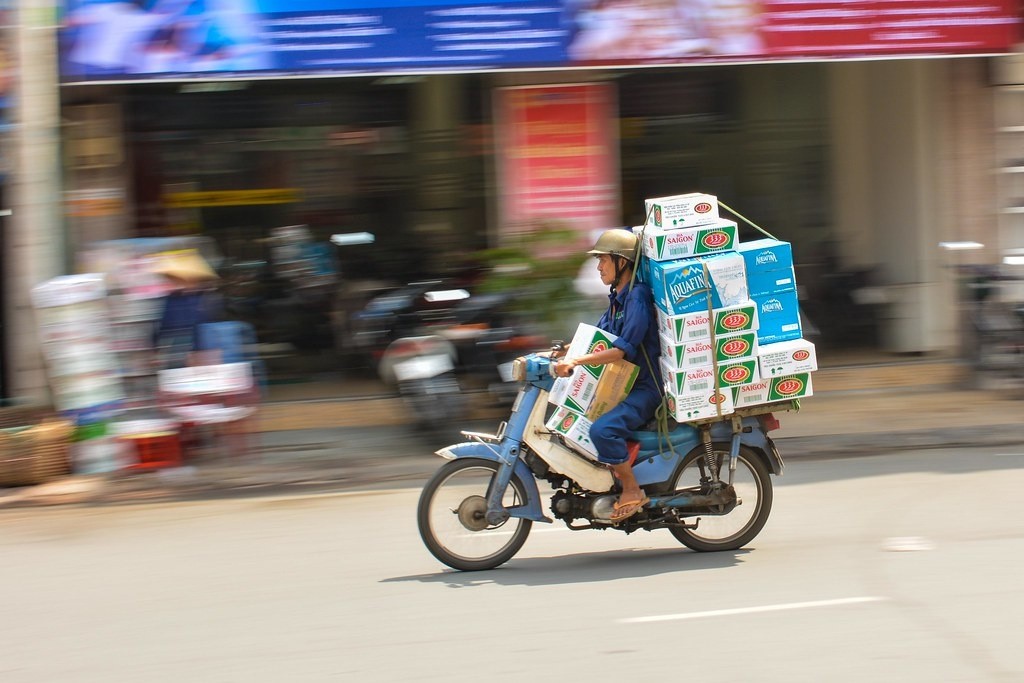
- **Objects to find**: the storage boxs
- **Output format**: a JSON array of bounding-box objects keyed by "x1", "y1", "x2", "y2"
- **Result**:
[{"x1": 0, "y1": 190, "x2": 820, "y2": 486}]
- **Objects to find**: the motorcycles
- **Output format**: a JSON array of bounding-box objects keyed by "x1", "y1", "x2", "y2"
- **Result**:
[
  {"x1": 952, "y1": 256, "x2": 1023, "y2": 392},
  {"x1": 419, "y1": 338, "x2": 784, "y2": 572}
]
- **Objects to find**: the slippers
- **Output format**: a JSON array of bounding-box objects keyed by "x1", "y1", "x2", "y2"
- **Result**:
[{"x1": 610, "y1": 497, "x2": 649, "y2": 521}]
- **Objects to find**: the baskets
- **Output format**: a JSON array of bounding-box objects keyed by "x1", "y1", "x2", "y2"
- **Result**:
[{"x1": 0, "y1": 419, "x2": 78, "y2": 487}]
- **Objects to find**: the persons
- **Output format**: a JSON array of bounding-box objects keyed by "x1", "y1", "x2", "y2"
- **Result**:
[{"x1": 516, "y1": 226, "x2": 663, "y2": 519}]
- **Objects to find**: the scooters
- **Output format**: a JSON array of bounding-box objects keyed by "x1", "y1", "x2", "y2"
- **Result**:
[
  {"x1": 376, "y1": 327, "x2": 465, "y2": 440},
  {"x1": 457, "y1": 284, "x2": 550, "y2": 416}
]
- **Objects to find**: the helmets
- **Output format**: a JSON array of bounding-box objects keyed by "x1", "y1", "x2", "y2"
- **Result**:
[{"x1": 585, "y1": 229, "x2": 641, "y2": 264}]
